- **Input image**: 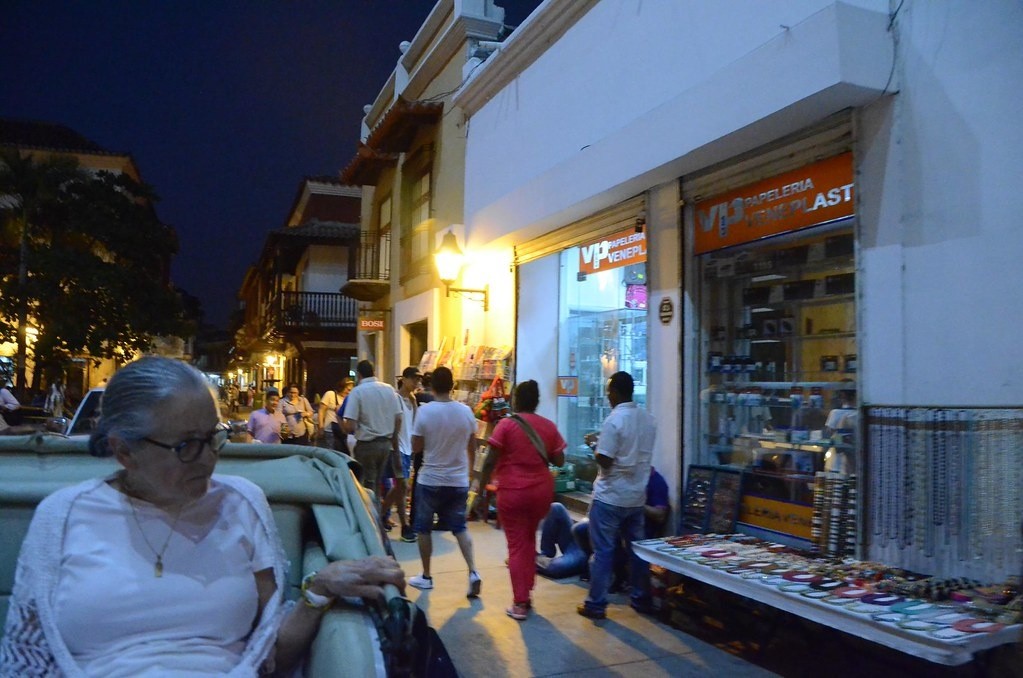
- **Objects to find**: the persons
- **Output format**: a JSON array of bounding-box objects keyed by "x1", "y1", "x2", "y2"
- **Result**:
[
  {"x1": 0, "y1": 374, "x2": 21, "y2": 434},
  {"x1": 700, "y1": 364, "x2": 857, "y2": 478},
  {"x1": 219, "y1": 360, "x2": 435, "y2": 542},
  {"x1": 505, "y1": 466, "x2": 671, "y2": 593},
  {"x1": 474, "y1": 380, "x2": 567, "y2": 621},
  {"x1": 408, "y1": 366, "x2": 481, "y2": 598},
  {"x1": 0, "y1": 357, "x2": 406, "y2": 678},
  {"x1": 577, "y1": 371, "x2": 657, "y2": 619}
]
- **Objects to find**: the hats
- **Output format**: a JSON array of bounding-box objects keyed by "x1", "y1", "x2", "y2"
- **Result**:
[
  {"x1": 403, "y1": 367, "x2": 425, "y2": 378},
  {"x1": 339, "y1": 377, "x2": 355, "y2": 387}
]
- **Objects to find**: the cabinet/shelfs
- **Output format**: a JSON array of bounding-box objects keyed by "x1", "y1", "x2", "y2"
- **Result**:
[
  {"x1": 565, "y1": 307, "x2": 646, "y2": 457},
  {"x1": 709, "y1": 258, "x2": 856, "y2": 447}
]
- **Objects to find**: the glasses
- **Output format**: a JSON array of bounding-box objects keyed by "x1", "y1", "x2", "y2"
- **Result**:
[{"x1": 142, "y1": 423, "x2": 229, "y2": 464}]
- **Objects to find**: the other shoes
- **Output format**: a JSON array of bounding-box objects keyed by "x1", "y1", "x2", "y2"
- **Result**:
[
  {"x1": 467, "y1": 574, "x2": 481, "y2": 597},
  {"x1": 506, "y1": 606, "x2": 527, "y2": 619},
  {"x1": 408, "y1": 573, "x2": 433, "y2": 588},
  {"x1": 577, "y1": 604, "x2": 607, "y2": 619},
  {"x1": 402, "y1": 528, "x2": 419, "y2": 542},
  {"x1": 384, "y1": 523, "x2": 392, "y2": 531}
]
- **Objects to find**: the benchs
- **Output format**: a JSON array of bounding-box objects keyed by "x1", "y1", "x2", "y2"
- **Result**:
[{"x1": 0, "y1": 488, "x2": 404, "y2": 678}]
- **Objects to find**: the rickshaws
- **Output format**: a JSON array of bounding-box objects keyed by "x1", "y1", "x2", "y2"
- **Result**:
[{"x1": 0, "y1": 434, "x2": 464, "y2": 677}]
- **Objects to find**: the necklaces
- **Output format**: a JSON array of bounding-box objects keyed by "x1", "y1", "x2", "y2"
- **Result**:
[
  {"x1": 126, "y1": 481, "x2": 181, "y2": 577},
  {"x1": 864, "y1": 405, "x2": 1023, "y2": 573},
  {"x1": 634, "y1": 533, "x2": 1023, "y2": 639}
]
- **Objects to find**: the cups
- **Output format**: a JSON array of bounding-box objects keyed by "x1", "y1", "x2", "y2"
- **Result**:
[
  {"x1": 774, "y1": 424, "x2": 823, "y2": 444},
  {"x1": 768, "y1": 285, "x2": 784, "y2": 302}
]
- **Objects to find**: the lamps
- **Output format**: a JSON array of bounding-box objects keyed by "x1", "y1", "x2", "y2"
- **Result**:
[{"x1": 429, "y1": 228, "x2": 492, "y2": 312}]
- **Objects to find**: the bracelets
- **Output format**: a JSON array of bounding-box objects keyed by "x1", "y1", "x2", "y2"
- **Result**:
[
  {"x1": 810, "y1": 477, "x2": 856, "y2": 559},
  {"x1": 301, "y1": 571, "x2": 336, "y2": 611},
  {"x1": 590, "y1": 442, "x2": 597, "y2": 451}
]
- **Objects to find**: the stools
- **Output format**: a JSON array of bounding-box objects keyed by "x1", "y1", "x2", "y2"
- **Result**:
[{"x1": 481, "y1": 482, "x2": 502, "y2": 530}]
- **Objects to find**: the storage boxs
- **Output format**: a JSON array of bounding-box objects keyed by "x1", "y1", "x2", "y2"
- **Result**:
[{"x1": 554, "y1": 463, "x2": 578, "y2": 492}]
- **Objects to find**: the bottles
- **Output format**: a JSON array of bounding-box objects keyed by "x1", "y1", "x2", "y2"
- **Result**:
[
  {"x1": 721, "y1": 358, "x2": 756, "y2": 371},
  {"x1": 790, "y1": 386, "x2": 803, "y2": 408},
  {"x1": 835, "y1": 429, "x2": 852, "y2": 444},
  {"x1": 809, "y1": 387, "x2": 824, "y2": 409},
  {"x1": 763, "y1": 317, "x2": 778, "y2": 335},
  {"x1": 719, "y1": 416, "x2": 737, "y2": 434},
  {"x1": 757, "y1": 361, "x2": 789, "y2": 382},
  {"x1": 708, "y1": 351, "x2": 722, "y2": 366},
  {"x1": 843, "y1": 354, "x2": 856, "y2": 373},
  {"x1": 819, "y1": 355, "x2": 838, "y2": 371},
  {"x1": 735, "y1": 309, "x2": 750, "y2": 338},
  {"x1": 719, "y1": 308, "x2": 728, "y2": 341},
  {"x1": 744, "y1": 307, "x2": 757, "y2": 338},
  {"x1": 726, "y1": 386, "x2": 762, "y2": 406},
  {"x1": 780, "y1": 315, "x2": 795, "y2": 333}
]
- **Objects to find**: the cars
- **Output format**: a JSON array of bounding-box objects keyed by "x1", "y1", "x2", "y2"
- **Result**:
[{"x1": 50, "y1": 388, "x2": 109, "y2": 436}]
